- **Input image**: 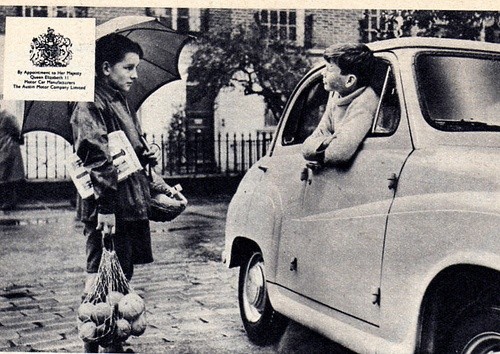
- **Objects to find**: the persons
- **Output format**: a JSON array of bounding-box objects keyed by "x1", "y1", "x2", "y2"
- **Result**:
[
  {"x1": 71, "y1": 32, "x2": 161, "y2": 353},
  {"x1": 302, "y1": 41, "x2": 382, "y2": 166}
]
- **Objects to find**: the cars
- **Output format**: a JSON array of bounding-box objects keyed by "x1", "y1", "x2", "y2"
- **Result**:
[{"x1": 221, "y1": 37, "x2": 500, "y2": 353}]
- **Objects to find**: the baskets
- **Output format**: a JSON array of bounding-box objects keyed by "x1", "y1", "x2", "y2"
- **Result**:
[{"x1": 147, "y1": 156, "x2": 188, "y2": 222}]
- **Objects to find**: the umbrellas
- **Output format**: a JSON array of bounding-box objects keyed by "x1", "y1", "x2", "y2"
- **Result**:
[{"x1": 22, "y1": 17, "x2": 202, "y2": 152}]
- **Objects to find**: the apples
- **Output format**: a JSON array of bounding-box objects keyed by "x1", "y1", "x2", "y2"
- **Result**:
[{"x1": 79, "y1": 291, "x2": 145, "y2": 346}]
